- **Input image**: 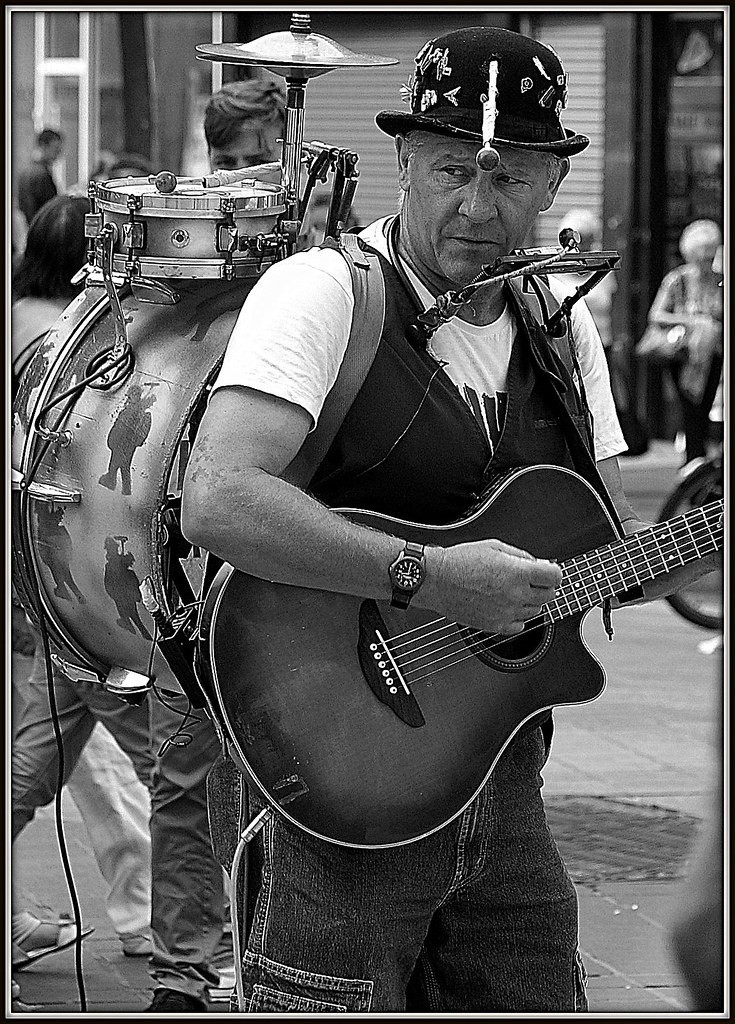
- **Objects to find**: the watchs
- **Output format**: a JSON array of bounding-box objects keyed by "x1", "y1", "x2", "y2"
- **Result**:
[{"x1": 388, "y1": 540, "x2": 426, "y2": 611}]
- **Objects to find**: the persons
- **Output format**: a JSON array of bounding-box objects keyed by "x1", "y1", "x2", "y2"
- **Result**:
[
  {"x1": 554, "y1": 206, "x2": 624, "y2": 370},
  {"x1": 8, "y1": 79, "x2": 358, "y2": 1011},
  {"x1": 650, "y1": 217, "x2": 723, "y2": 460},
  {"x1": 179, "y1": 26, "x2": 657, "y2": 1014}
]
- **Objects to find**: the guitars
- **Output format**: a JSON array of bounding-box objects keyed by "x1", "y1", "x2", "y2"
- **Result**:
[{"x1": 197, "y1": 465, "x2": 725, "y2": 855}]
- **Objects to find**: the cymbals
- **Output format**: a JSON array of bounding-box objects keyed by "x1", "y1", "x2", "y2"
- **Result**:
[{"x1": 190, "y1": 27, "x2": 400, "y2": 78}]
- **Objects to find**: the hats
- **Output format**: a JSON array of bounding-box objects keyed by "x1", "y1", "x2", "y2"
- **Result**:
[{"x1": 375, "y1": 26, "x2": 590, "y2": 155}]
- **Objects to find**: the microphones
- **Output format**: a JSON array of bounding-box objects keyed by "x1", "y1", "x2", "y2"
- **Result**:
[{"x1": 453, "y1": 229, "x2": 582, "y2": 301}]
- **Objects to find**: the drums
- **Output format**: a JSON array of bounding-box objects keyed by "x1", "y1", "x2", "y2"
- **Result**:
[{"x1": 17, "y1": 175, "x2": 299, "y2": 703}]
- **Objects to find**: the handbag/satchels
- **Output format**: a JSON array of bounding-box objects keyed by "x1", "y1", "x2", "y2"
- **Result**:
[{"x1": 635, "y1": 271, "x2": 690, "y2": 365}]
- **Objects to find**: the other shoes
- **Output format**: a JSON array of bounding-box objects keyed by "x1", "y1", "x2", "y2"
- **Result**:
[{"x1": 143, "y1": 988, "x2": 207, "y2": 1013}]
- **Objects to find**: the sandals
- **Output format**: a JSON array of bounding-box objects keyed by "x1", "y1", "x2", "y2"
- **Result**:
[
  {"x1": 119, "y1": 934, "x2": 153, "y2": 957},
  {"x1": 12, "y1": 911, "x2": 96, "y2": 970}
]
